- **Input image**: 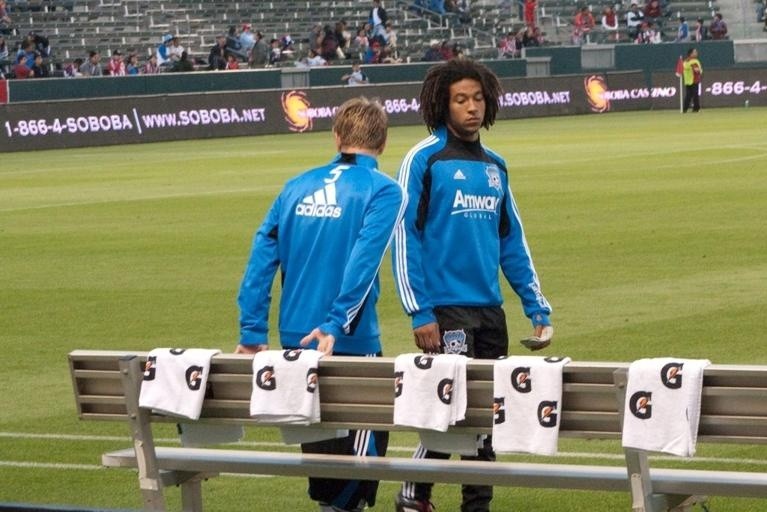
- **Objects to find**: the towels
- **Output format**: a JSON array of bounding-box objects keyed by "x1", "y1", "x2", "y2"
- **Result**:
[
  {"x1": 392, "y1": 352, "x2": 484, "y2": 458},
  {"x1": 139, "y1": 348, "x2": 244, "y2": 447},
  {"x1": 621, "y1": 355, "x2": 711, "y2": 458},
  {"x1": 250, "y1": 349, "x2": 349, "y2": 446},
  {"x1": 492, "y1": 355, "x2": 571, "y2": 456}
]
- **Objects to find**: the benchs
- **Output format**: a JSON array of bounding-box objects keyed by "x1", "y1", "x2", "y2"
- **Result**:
[{"x1": 67, "y1": 348, "x2": 767, "y2": 512}]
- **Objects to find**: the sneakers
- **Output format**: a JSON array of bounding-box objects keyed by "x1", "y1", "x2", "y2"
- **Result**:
[{"x1": 394, "y1": 494, "x2": 435, "y2": 512}]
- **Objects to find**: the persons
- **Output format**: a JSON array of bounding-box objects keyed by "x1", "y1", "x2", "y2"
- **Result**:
[
  {"x1": 64, "y1": 34, "x2": 196, "y2": 77},
  {"x1": 234, "y1": 94, "x2": 412, "y2": 512},
  {"x1": 754, "y1": 0, "x2": 767, "y2": 23},
  {"x1": 501, "y1": 26, "x2": 550, "y2": 52},
  {"x1": 678, "y1": 14, "x2": 724, "y2": 41},
  {"x1": 680, "y1": 46, "x2": 703, "y2": 114},
  {"x1": 368, "y1": 0, "x2": 387, "y2": 36},
  {"x1": 0, "y1": 0, "x2": 51, "y2": 79},
  {"x1": 420, "y1": 0, "x2": 472, "y2": 21},
  {"x1": 208, "y1": 21, "x2": 398, "y2": 71},
  {"x1": 388, "y1": 58, "x2": 556, "y2": 511},
  {"x1": 425, "y1": 39, "x2": 464, "y2": 62},
  {"x1": 574, "y1": 0, "x2": 664, "y2": 44},
  {"x1": 342, "y1": 60, "x2": 368, "y2": 85}
]
